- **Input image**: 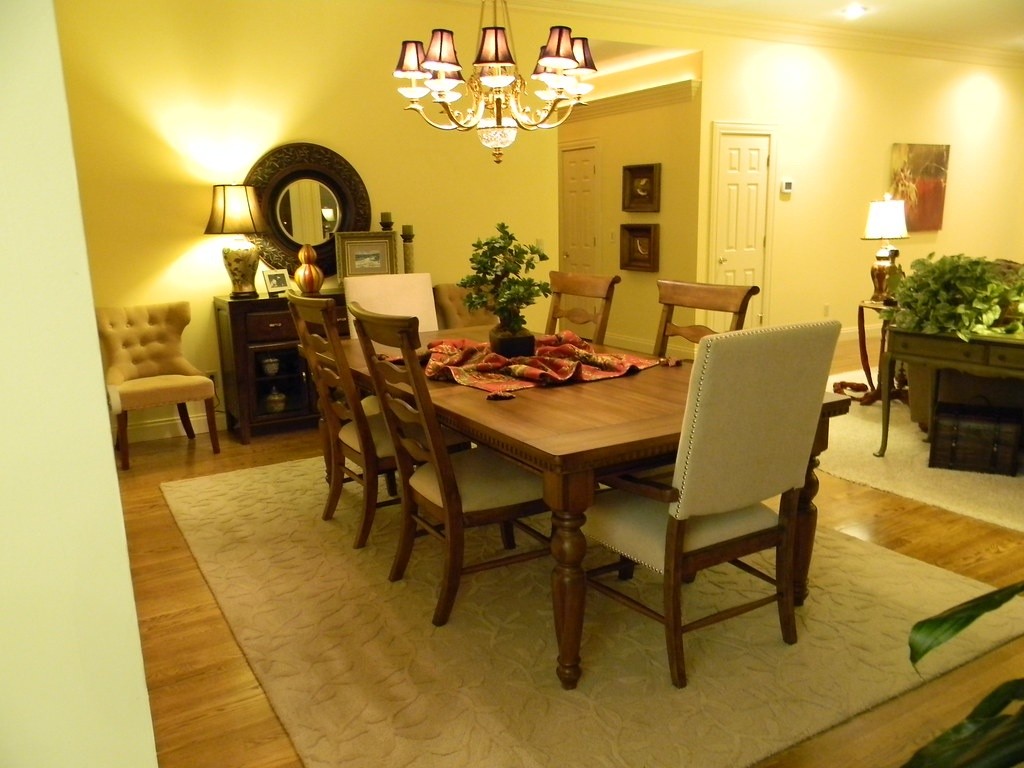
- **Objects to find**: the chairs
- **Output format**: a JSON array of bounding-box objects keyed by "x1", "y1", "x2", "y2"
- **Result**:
[
  {"x1": 94, "y1": 300, "x2": 220, "y2": 471},
  {"x1": 345, "y1": 273, "x2": 439, "y2": 342},
  {"x1": 544, "y1": 270, "x2": 759, "y2": 585},
  {"x1": 432, "y1": 282, "x2": 500, "y2": 330},
  {"x1": 285, "y1": 294, "x2": 552, "y2": 626},
  {"x1": 580, "y1": 318, "x2": 843, "y2": 688}
]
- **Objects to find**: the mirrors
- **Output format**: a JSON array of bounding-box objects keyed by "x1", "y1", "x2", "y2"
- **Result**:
[{"x1": 242, "y1": 142, "x2": 372, "y2": 281}]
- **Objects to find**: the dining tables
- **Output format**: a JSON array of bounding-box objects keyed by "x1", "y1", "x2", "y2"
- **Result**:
[{"x1": 298, "y1": 320, "x2": 849, "y2": 692}]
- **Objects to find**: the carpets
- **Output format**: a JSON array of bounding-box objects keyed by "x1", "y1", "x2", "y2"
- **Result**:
[
  {"x1": 160, "y1": 450, "x2": 1024, "y2": 768},
  {"x1": 871, "y1": 325, "x2": 1023, "y2": 457}
]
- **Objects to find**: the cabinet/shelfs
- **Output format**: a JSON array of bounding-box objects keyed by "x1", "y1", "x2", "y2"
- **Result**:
[{"x1": 214, "y1": 296, "x2": 349, "y2": 446}]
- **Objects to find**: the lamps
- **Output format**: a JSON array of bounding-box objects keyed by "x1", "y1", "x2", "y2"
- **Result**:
[
  {"x1": 392, "y1": 1, "x2": 599, "y2": 164},
  {"x1": 860, "y1": 193, "x2": 910, "y2": 303},
  {"x1": 202, "y1": 183, "x2": 269, "y2": 301}
]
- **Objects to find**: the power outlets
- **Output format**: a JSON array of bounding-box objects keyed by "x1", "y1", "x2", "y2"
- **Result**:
[{"x1": 206, "y1": 370, "x2": 218, "y2": 389}]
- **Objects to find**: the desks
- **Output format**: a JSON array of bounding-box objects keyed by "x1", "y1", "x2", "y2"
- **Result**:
[{"x1": 858, "y1": 299, "x2": 911, "y2": 411}]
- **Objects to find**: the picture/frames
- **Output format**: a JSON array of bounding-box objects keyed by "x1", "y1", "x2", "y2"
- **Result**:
[
  {"x1": 336, "y1": 231, "x2": 398, "y2": 290},
  {"x1": 263, "y1": 269, "x2": 290, "y2": 292}
]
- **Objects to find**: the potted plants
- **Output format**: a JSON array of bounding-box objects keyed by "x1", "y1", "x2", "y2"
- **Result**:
[
  {"x1": 457, "y1": 222, "x2": 554, "y2": 357},
  {"x1": 878, "y1": 251, "x2": 1024, "y2": 342}
]
